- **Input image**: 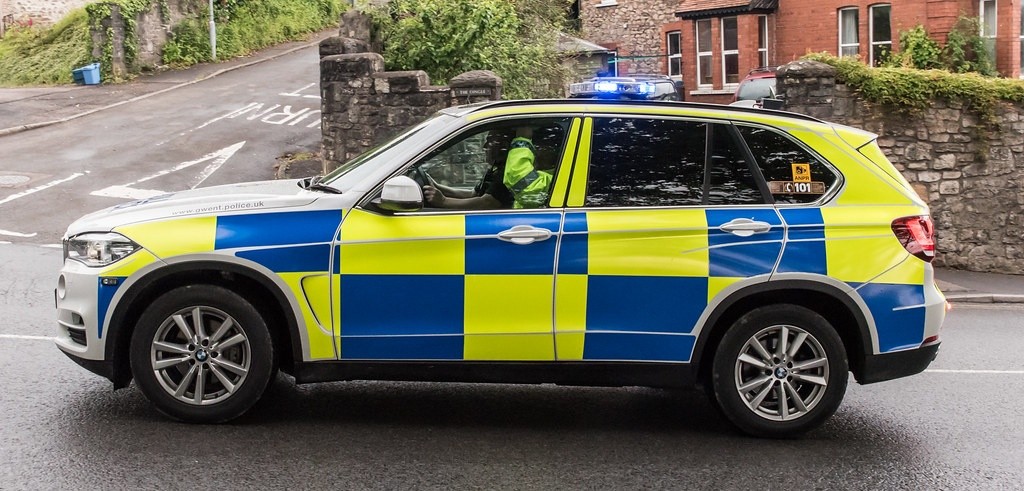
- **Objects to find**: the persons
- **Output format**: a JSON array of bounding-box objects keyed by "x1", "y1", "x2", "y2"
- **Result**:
[{"x1": 424, "y1": 125, "x2": 565, "y2": 209}]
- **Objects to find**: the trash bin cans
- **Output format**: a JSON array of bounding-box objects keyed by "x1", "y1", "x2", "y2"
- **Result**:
[
  {"x1": 81, "y1": 62, "x2": 101, "y2": 85},
  {"x1": 72, "y1": 67, "x2": 85, "y2": 86}
]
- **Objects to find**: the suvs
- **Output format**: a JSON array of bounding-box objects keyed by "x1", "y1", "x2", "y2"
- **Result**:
[
  {"x1": 731, "y1": 66, "x2": 776, "y2": 102},
  {"x1": 54, "y1": 70, "x2": 947, "y2": 440},
  {"x1": 627, "y1": 74, "x2": 685, "y2": 101}
]
